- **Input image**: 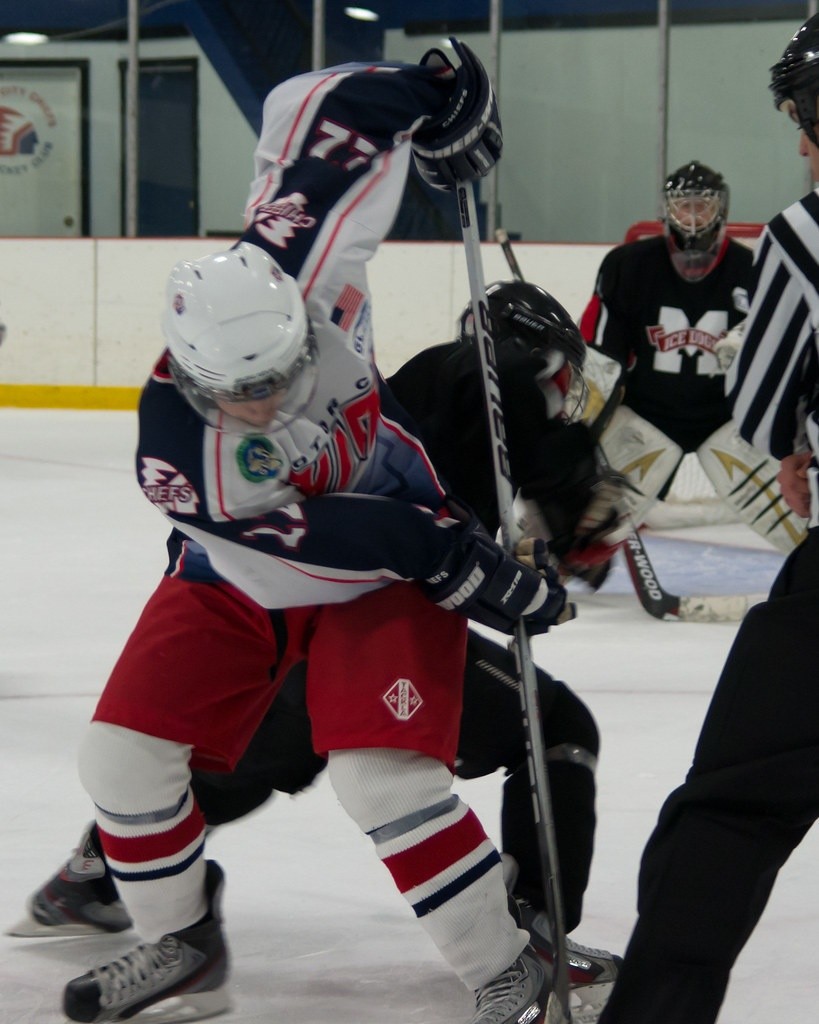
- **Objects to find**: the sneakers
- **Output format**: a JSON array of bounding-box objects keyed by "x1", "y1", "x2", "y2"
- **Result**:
[
  {"x1": 461, "y1": 893, "x2": 565, "y2": 1023},
  {"x1": 61, "y1": 860, "x2": 233, "y2": 1023},
  {"x1": 10, "y1": 823, "x2": 134, "y2": 940},
  {"x1": 503, "y1": 854, "x2": 625, "y2": 1023}
]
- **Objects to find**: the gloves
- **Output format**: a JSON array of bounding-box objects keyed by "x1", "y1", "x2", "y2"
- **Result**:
[
  {"x1": 420, "y1": 497, "x2": 576, "y2": 638},
  {"x1": 413, "y1": 36, "x2": 504, "y2": 194}
]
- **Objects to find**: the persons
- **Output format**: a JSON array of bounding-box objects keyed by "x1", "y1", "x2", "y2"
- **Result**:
[
  {"x1": 57, "y1": 63, "x2": 569, "y2": 1024},
  {"x1": 578, "y1": 154, "x2": 814, "y2": 602},
  {"x1": 603, "y1": 11, "x2": 819, "y2": 1022},
  {"x1": 23, "y1": 277, "x2": 632, "y2": 993}
]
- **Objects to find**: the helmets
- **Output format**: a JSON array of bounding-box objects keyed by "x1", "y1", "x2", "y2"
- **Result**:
[
  {"x1": 765, "y1": 13, "x2": 819, "y2": 146},
  {"x1": 460, "y1": 280, "x2": 589, "y2": 424},
  {"x1": 158, "y1": 246, "x2": 317, "y2": 435},
  {"x1": 661, "y1": 165, "x2": 730, "y2": 257}
]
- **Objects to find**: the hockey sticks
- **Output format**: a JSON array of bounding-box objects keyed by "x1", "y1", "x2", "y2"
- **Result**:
[{"x1": 459, "y1": 181, "x2": 746, "y2": 1024}]
